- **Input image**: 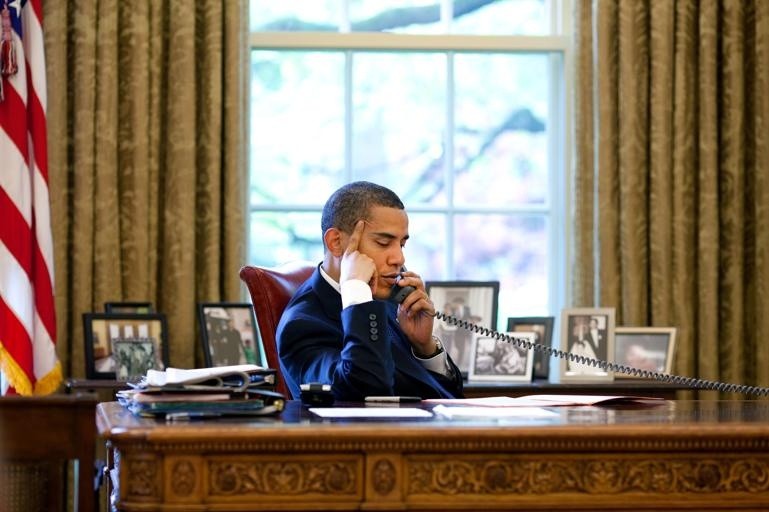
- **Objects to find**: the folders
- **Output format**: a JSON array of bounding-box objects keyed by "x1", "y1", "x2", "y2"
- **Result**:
[{"x1": 116, "y1": 363, "x2": 286, "y2": 418}]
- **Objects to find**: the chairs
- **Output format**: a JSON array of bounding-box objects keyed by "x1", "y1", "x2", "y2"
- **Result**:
[{"x1": 239, "y1": 265, "x2": 317, "y2": 400}]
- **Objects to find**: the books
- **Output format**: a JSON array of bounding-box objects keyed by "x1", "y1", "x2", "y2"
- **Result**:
[{"x1": 114, "y1": 364, "x2": 287, "y2": 421}]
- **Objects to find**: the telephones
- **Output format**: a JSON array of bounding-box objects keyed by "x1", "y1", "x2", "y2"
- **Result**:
[{"x1": 390, "y1": 265, "x2": 416, "y2": 303}]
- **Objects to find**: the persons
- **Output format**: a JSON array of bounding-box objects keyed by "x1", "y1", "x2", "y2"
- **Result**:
[
  {"x1": 115, "y1": 342, "x2": 159, "y2": 380},
  {"x1": 440, "y1": 296, "x2": 482, "y2": 370},
  {"x1": 566, "y1": 318, "x2": 606, "y2": 376},
  {"x1": 274, "y1": 180, "x2": 466, "y2": 408},
  {"x1": 206, "y1": 311, "x2": 261, "y2": 366},
  {"x1": 477, "y1": 338, "x2": 528, "y2": 378}
]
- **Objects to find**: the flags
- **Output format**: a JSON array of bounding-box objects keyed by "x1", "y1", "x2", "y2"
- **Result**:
[{"x1": 0, "y1": 0, "x2": 64, "y2": 400}]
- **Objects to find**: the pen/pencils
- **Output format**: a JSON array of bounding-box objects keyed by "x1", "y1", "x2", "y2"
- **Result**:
[
  {"x1": 165, "y1": 412, "x2": 222, "y2": 420},
  {"x1": 365, "y1": 396, "x2": 422, "y2": 403}
]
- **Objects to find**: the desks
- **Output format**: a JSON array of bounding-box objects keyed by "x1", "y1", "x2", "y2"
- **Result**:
[
  {"x1": 462, "y1": 378, "x2": 687, "y2": 399},
  {"x1": 91, "y1": 396, "x2": 769, "y2": 512}
]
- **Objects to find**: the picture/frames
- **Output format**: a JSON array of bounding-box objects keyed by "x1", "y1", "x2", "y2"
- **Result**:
[
  {"x1": 104, "y1": 302, "x2": 153, "y2": 314},
  {"x1": 614, "y1": 326, "x2": 677, "y2": 379},
  {"x1": 559, "y1": 307, "x2": 617, "y2": 381},
  {"x1": 425, "y1": 279, "x2": 500, "y2": 380},
  {"x1": 81, "y1": 312, "x2": 169, "y2": 380},
  {"x1": 197, "y1": 302, "x2": 262, "y2": 368},
  {"x1": 467, "y1": 331, "x2": 536, "y2": 383},
  {"x1": 112, "y1": 337, "x2": 157, "y2": 382},
  {"x1": 507, "y1": 317, "x2": 555, "y2": 378}
]
